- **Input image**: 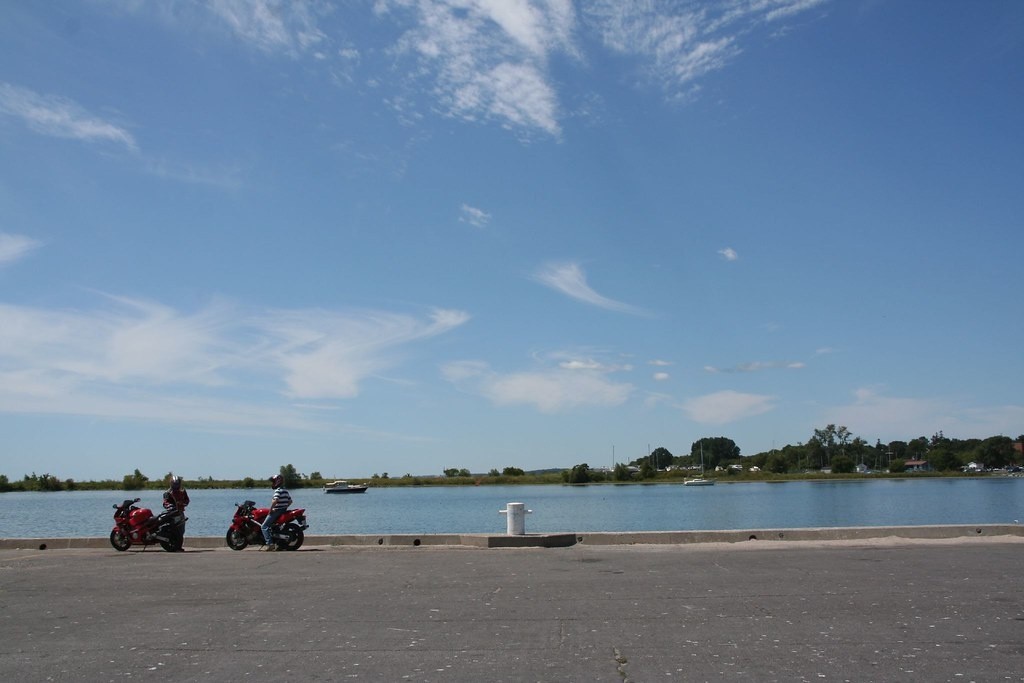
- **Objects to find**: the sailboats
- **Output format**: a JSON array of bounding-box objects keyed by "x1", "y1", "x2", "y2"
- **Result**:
[{"x1": 684, "y1": 442, "x2": 715, "y2": 486}]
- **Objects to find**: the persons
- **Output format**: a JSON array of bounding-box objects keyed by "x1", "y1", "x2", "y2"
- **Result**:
[
  {"x1": 261, "y1": 475, "x2": 293, "y2": 551},
  {"x1": 163, "y1": 476, "x2": 190, "y2": 550}
]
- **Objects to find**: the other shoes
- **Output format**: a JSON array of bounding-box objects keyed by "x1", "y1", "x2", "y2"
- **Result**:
[
  {"x1": 266, "y1": 545, "x2": 275, "y2": 551},
  {"x1": 178, "y1": 547, "x2": 184, "y2": 552}
]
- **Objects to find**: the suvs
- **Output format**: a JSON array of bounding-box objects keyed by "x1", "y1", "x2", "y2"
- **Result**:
[{"x1": 1007, "y1": 466, "x2": 1019, "y2": 472}]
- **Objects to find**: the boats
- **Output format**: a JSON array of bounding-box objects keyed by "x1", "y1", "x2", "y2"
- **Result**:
[{"x1": 324, "y1": 481, "x2": 368, "y2": 494}]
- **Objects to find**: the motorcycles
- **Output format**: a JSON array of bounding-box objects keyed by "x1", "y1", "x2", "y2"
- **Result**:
[
  {"x1": 109, "y1": 497, "x2": 189, "y2": 552},
  {"x1": 225, "y1": 500, "x2": 310, "y2": 551}
]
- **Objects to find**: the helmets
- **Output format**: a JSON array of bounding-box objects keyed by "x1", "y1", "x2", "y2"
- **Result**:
[
  {"x1": 170, "y1": 476, "x2": 181, "y2": 490},
  {"x1": 269, "y1": 475, "x2": 283, "y2": 489}
]
